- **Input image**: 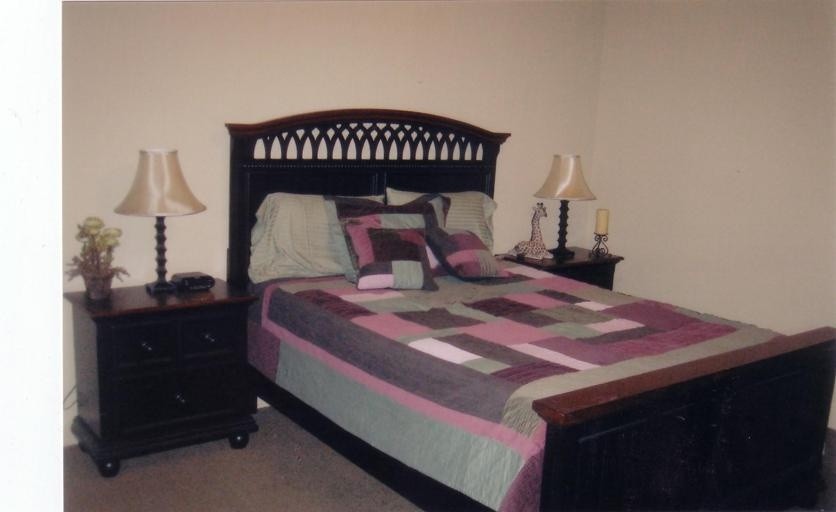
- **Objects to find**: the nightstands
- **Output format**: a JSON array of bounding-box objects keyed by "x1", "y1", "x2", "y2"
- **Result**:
[
  {"x1": 502, "y1": 245, "x2": 625, "y2": 293},
  {"x1": 65, "y1": 274, "x2": 257, "y2": 478}
]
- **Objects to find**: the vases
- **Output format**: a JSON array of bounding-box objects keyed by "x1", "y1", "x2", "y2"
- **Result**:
[{"x1": 81, "y1": 270, "x2": 112, "y2": 300}]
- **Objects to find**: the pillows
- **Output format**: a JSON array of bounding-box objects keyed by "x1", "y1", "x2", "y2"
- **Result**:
[
  {"x1": 333, "y1": 191, "x2": 452, "y2": 277},
  {"x1": 346, "y1": 222, "x2": 439, "y2": 291},
  {"x1": 249, "y1": 193, "x2": 385, "y2": 283},
  {"x1": 384, "y1": 188, "x2": 498, "y2": 262},
  {"x1": 435, "y1": 227, "x2": 518, "y2": 282}
]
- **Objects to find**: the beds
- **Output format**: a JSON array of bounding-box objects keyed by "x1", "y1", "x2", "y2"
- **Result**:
[{"x1": 225, "y1": 107, "x2": 835, "y2": 512}]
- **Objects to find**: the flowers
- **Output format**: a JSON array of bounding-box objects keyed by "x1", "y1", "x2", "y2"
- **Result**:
[{"x1": 65, "y1": 206, "x2": 134, "y2": 283}]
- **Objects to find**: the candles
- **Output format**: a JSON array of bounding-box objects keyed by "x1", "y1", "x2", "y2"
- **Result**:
[{"x1": 594, "y1": 208, "x2": 609, "y2": 236}]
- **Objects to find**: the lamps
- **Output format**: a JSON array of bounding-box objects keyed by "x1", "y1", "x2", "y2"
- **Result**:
[
  {"x1": 116, "y1": 146, "x2": 206, "y2": 296},
  {"x1": 532, "y1": 153, "x2": 594, "y2": 257}
]
